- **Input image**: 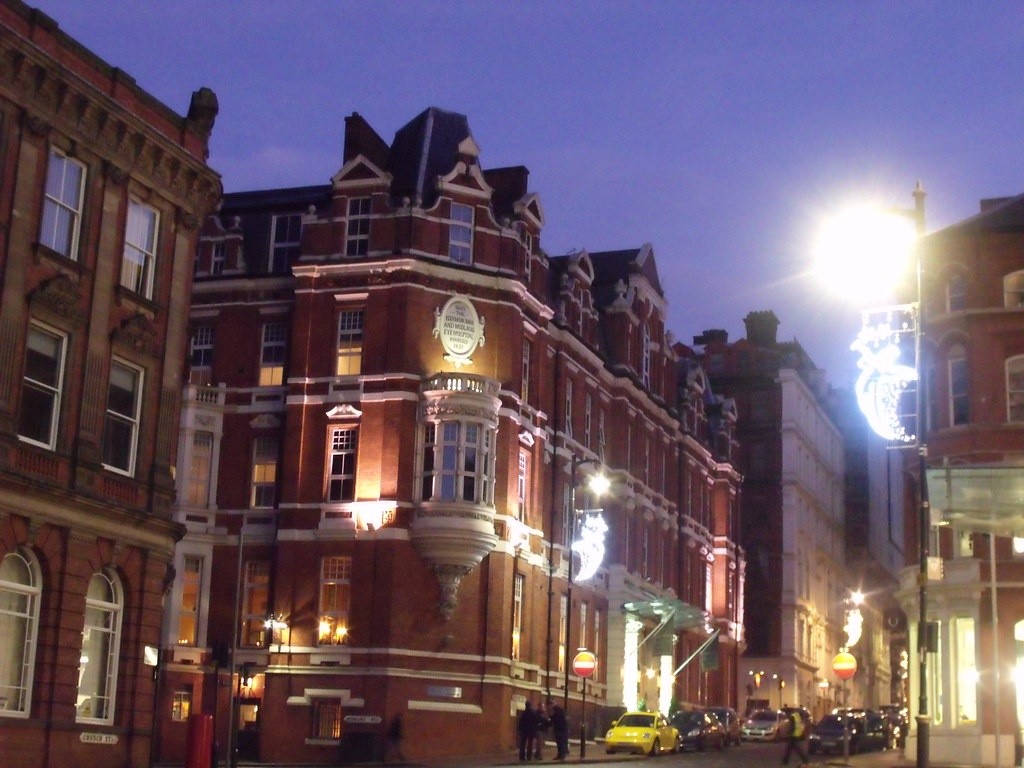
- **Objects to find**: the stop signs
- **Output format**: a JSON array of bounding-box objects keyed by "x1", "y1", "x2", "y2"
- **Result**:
[{"x1": 574, "y1": 653, "x2": 597, "y2": 677}]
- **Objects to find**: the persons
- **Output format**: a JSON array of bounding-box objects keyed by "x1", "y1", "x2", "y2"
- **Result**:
[
  {"x1": 783, "y1": 709, "x2": 809, "y2": 765},
  {"x1": 381, "y1": 713, "x2": 406, "y2": 761},
  {"x1": 517, "y1": 699, "x2": 570, "y2": 760}
]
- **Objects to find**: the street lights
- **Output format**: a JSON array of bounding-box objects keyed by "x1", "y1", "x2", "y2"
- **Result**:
[
  {"x1": 561, "y1": 448, "x2": 610, "y2": 753},
  {"x1": 848, "y1": 184, "x2": 933, "y2": 768}
]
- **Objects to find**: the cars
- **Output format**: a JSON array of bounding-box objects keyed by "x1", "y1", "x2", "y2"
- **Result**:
[
  {"x1": 604, "y1": 712, "x2": 681, "y2": 755},
  {"x1": 702, "y1": 708, "x2": 742, "y2": 746},
  {"x1": 809, "y1": 705, "x2": 909, "y2": 754},
  {"x1": 672, "y1": 713, "x2": 724, "y2": 751},
  {"x1": 781, "y1": 707, "x2": 814, "y2": 740},
  {"x1": 741, "y1": 708, "x2": 790, "y2": 742}
]
